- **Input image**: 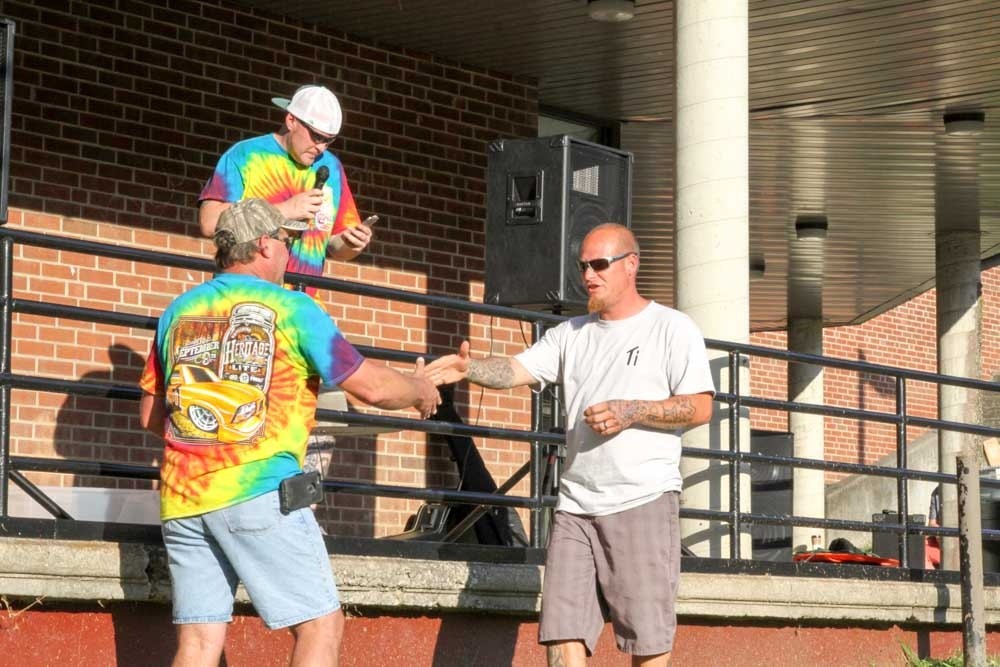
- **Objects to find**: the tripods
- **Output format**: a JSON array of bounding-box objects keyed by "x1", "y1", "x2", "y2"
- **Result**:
[{"x1": 441, "y1": 384, "x2": 695, "y2": 559}]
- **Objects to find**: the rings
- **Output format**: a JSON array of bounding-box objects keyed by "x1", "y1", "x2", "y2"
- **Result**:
[{"x1": 604, "y1": 421, "x2": 608, "y2": 429}]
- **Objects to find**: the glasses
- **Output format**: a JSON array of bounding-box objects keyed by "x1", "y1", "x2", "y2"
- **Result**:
[
  {"x1": 296, "y1": 118, "x2": 337, "y2": 145},
  {"x1": 575, "y1": 251, "x2": 637, "y2": 273}
]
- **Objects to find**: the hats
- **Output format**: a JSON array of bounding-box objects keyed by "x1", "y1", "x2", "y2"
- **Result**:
[
  {"x1": 271, "y1": 85, "x2": 342, "y2": 135},
  {"x1": 214, "y1": 199, "x2": 309, "y2": 243}
]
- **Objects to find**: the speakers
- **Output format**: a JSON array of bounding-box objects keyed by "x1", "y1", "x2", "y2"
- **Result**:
[
  {"x1": 484, "y1": 135, "x2": 634, "y2": 314},
  {"x1": 872, "y1": 513, "x2": 926, "y2": 570},
  {"x1": 752, "y1": 429, "x2": 795, "y2": 560}
]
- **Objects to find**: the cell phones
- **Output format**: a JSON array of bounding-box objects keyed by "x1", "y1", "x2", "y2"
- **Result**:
[{"x1": 361, "y1": 216, "x2": 379, "y2": 226}]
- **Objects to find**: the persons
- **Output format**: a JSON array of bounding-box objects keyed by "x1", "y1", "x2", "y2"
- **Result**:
[
  {"x1": 425, "y1": 221, "x2": 717, "y2": 667},
  {"x1": 197, "y1": 83, "x2": 372, "y2": 310},
  {"x1": 928, "y1": 485, "x2": 943, "y2": 569},
  {"x1": 830, "y1": 537, "x2": 862, "y2": 554},
  {"x1": 138, "y1": 200, "x2": 442, "y2": 667}
]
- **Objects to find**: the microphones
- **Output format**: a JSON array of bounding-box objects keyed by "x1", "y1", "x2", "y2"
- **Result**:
[{"x1": 298, "y1": 166, "x2": 331, "y2": 237}]
